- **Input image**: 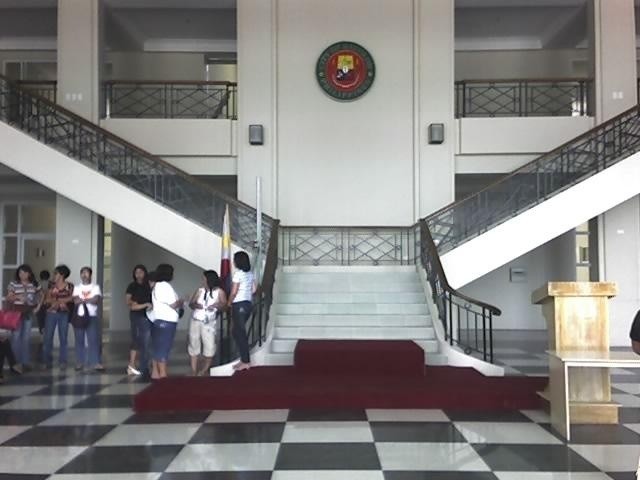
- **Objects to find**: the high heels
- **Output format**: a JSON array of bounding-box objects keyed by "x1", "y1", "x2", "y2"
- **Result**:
[
  {"x1": 234, "y1": 361, "x2": 250, "y2": 371},
  {"x1": 127, "y1": 365, "x2": 143, "y2": 375}
]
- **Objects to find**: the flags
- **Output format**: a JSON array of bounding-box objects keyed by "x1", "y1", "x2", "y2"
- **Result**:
[{"x1": 222, "y1": 208, "x2": 231, "y2": 296}]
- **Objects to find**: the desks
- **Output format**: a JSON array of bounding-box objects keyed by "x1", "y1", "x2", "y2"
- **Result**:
[{"x1": 545, "y1": 350, "x2": 640, "y2": 441}]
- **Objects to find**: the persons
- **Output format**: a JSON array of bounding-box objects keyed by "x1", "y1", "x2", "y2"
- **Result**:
[
  {"x1": 5, "y1": 264, "x2": 186, "y2": 380},
  {"x1": 227, "y1": 251, "x2": 256, "y2": 369},
  {"x1": 186, "y1": 270, "x2": 227, "y2": 378}
]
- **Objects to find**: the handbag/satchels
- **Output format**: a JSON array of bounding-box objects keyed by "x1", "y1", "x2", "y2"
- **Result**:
[
  {"x1": 71, "y1": 303, "x2": 88, "y2": 328},
  {"x1": 0, "y1": 310, "x2": 22, "y2": 329}
]
